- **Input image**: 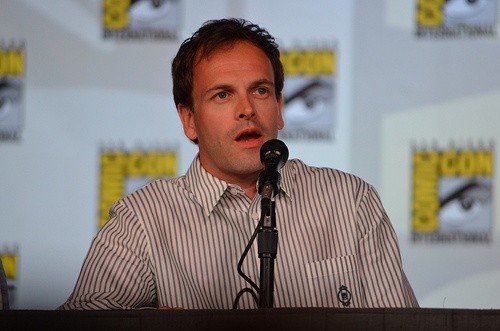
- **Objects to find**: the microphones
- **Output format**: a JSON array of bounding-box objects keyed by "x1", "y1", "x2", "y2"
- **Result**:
[{"x1": 260, "y1": 139, "x2": 290, "y2": 210}]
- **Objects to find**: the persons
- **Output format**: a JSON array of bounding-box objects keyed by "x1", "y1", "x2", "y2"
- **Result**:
[{"x1": 57, "y1": 18, "x2": 419, "y2": 308}]
